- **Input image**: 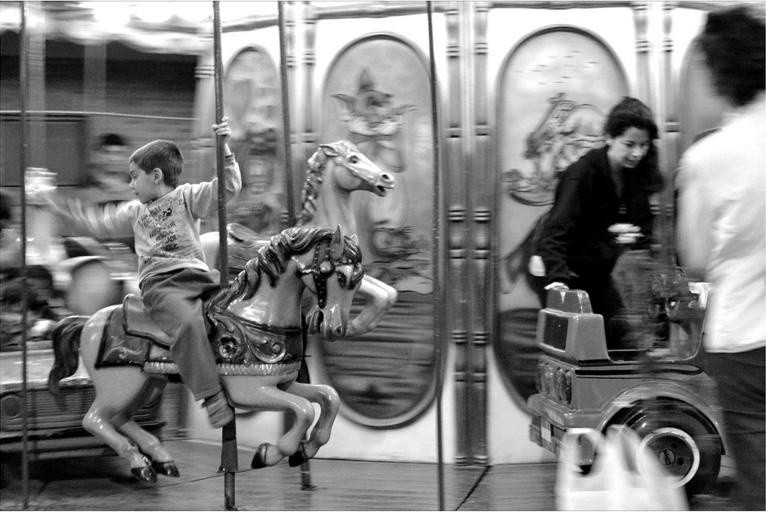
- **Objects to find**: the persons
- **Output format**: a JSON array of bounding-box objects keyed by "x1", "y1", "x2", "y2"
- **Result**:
[
  {"x1": 676, "y1": 6, "x2": 766, "y2": 511},
  {"x1": 518, "y1": 96, "x2": 673, "y2": 359},
  {"x1": 0, "y1": 117, "x2": 242, "y2": 430}
]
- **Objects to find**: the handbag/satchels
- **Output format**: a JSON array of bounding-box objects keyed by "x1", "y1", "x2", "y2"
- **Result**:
[{"x1": 558, "y1": 424, "x2": 690, "y2": 510}]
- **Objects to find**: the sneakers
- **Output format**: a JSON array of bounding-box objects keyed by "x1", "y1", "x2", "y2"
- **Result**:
[{"x1": 203, "y1": 392, "x2": 232, "y2": 427}]
